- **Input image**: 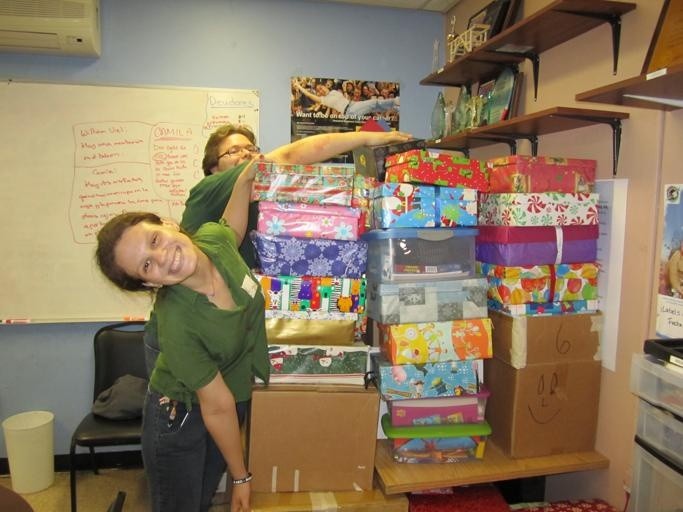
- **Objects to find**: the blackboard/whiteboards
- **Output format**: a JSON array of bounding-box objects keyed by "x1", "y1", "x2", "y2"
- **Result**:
[{"x1": 0, "y1": 81, "x2": 260, "y2": 326}]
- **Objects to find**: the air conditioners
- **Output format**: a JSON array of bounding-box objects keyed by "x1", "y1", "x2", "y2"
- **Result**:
[{"x1": 0, "y1": 0, "x2": 102, "y2": 58}]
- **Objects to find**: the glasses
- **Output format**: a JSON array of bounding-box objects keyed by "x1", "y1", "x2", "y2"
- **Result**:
[{"x1": 217, "y1": 144, "x2": 260, "y2": 160}]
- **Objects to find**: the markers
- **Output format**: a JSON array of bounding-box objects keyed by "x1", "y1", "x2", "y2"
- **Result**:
[
  {"x1": 123, "y1": 314, "x2": 145, "y2": 321},
  {"x1": 0, "y1": 319, "x2": 31, "y2": 324}
]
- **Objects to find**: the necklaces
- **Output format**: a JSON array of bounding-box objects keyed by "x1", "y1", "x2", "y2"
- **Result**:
[{"x1": 207, "y1": 270, "x2": 216, "y2": 296}]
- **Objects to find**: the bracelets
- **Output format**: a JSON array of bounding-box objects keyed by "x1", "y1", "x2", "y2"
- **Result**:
[{"x1": 231, "y1": 471, "x2": 253, "y2": 484}]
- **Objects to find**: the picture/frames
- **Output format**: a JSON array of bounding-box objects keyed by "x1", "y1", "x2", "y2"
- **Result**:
[
  {"x1": 501, "y1": 0, "x2": 519, "y2": 33},
  {"x1": 476, "y1": 72, "x2": 523, "y2": 120},
  {"x1": 467, "y1": 0, "x2": 510, "y2": 40},
  {"x1": 640, "y1": 0, "x2": 683, "y2": 74}
]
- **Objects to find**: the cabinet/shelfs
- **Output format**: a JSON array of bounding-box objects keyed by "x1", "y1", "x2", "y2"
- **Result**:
[{"x1": 419, "y1": 0, "x2": 683, "y2": 174}]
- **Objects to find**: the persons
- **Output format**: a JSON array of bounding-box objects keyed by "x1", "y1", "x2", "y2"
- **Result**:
[
  {"x1": 290, "y1": 77, "x2": 398, "y2": 122},
  {"x1": 143, "y1": 127, "x2": 411, "y2": 378},
  {"x1": 94, "y1": 153, "x2": 269, "y2": 511}
]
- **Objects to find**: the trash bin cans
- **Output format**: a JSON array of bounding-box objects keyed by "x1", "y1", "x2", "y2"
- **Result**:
[{"x1": 1, "y1": 410, "x2": 55, "y2": 494}]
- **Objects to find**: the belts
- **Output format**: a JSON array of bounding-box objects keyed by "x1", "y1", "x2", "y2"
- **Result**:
[{"x1": 343, "y1": 102, "x2": 350, "y2": 114}]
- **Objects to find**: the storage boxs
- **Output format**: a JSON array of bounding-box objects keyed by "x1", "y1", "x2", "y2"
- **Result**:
[
  {"x1": 362, "y1": 136, "x2": 493, "y2": 467},
  {"x1": 471, "y1": 137, "x2": 605, "y2": 459},
  {"x1": 238, "y1": 137, "x2": 380, "y2": 494}
]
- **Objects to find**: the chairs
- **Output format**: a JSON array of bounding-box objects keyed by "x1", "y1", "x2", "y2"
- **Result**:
[{"x1": 70, "y1": 321, "x2": 149, "y2": 512}]
